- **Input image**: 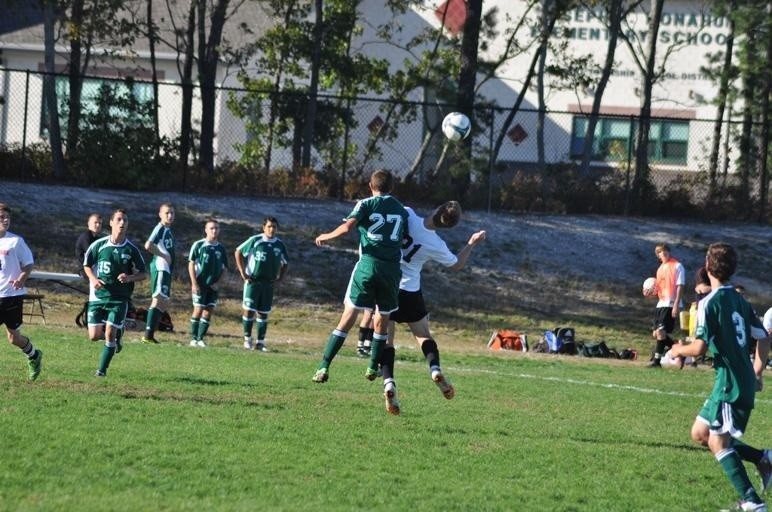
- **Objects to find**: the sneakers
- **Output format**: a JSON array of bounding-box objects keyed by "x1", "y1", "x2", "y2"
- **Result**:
[
  {"x1": 255, "y1": 343, "x2": 267, "y2": 352},
  {"x1": 646, "y1": 360, "x2": 662, "y2": 369},
  {"x1": 141, "y1": 336, "x2": 160, "y2": 345},
  {"x1": 755, "y1": 451, "x2": 772, "y2": 494},
  {"x1": 384, "y1": 382, "x2": 401, "y2": 417},
  {"x1": 356, "y1": 346, "x2": 373, "y2": 359},
  {"x1": 244, "y1": 341, "x2": 254, "y2": 351},
  {"x1": 720, "y1": 501, "x2": 767, "y2": 512},
  {"x1": 365, "y1": 367, "x2": 383, "y2": 382},
  {"x1": 312, "y1": 367, "x2": 329, "y2": 384},
  {"x1": 190, "y1": 340, "x2": 206, "y2": 348},
  {"x1": 431, "y1": 371, "x2": 455, "y2": 401},
  {"x1": 27, "y1": 349, "x2": 42, "y2": 382},
  {"x1": 114, "y1": 325, "x2": 125, "y2": 354}
]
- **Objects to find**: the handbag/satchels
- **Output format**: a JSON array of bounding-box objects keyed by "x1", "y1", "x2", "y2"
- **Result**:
[
  {"x1": 487, "y1": 329, "x2": 529, "y2": 353},
  {"x1": 533, "y1": 326, "x2": 638, "y2": 360},
  {"x1": 74, "y1": 301, "x2": 174, "y2": 332}
]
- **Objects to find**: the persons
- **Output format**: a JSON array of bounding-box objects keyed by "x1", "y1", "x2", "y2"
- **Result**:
[
  {"x1": 383, "y1": 199, "x2": 488, "y2": 416},
  {"x1": 235, "y1": 216, "x2": 288, "y2": 352},
  {"x1": 313, "y1": 167, "x2": 412, "y2": 416},
  {"x1": 686, "y1": 255, "x2": 772, "y2": 368},
  {"x1": 82, "y1": 207, "x2": 149, "y2": 376},
  {"x1": 0, "y1": 204, "x2": 44, "y2": 383},
  {"x1": 145, "y1": 201, "x2": 182, "y2": 345},
  {"x1": 657, "y1": 243, "x2": 772, "y2": 512},
  {"x1": 639, "y1": 243, "x2": 686, "y2": 371},
  {"x1": 354, "y1": 301, "x2": 380, "y2": 358},
  {"x1": 75, "y1": 214, "x2": 109, "y2": 332},
  {"x1": 188, "y1": 219, "x2": 230, "y2": 348}
]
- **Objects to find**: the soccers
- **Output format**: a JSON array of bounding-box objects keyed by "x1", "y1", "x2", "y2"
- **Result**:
[
  {"x1": 440, "y1": 111, "x2": 472, "y2": 142},
  {"x1": 642, "y1": 276, "x2": 658, "y2": 297}
]
